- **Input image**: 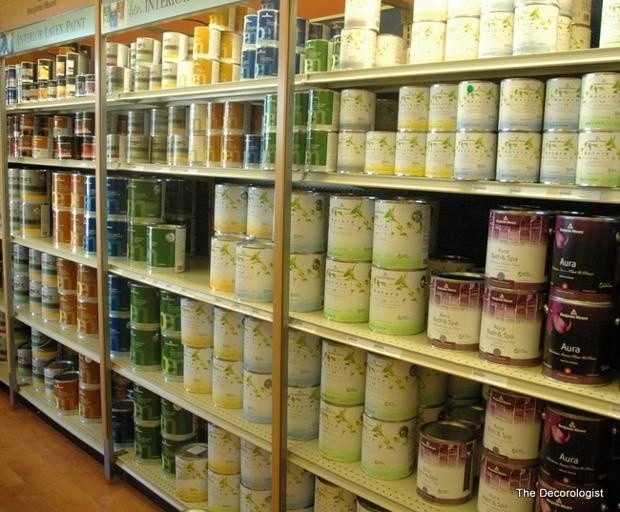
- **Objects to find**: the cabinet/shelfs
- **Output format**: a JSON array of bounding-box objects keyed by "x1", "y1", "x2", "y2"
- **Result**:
[{"x1": 0, "y1": 0, "x2": 619, "y2": 509}]
